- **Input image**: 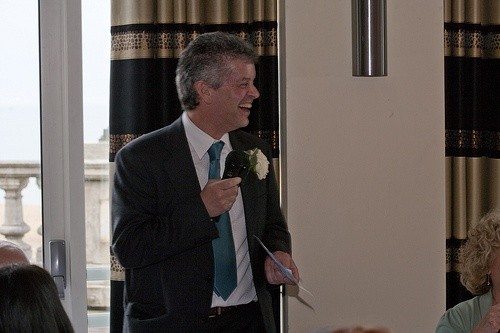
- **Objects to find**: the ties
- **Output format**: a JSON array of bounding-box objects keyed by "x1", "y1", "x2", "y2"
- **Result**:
[{"x1": 207, "y1": 141, "x2": 237, "y2": 300}]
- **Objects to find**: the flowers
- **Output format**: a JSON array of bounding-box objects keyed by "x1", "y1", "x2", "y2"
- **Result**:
[{"x1": 244, "y1": 148, "x2": 270, "y2": 180}]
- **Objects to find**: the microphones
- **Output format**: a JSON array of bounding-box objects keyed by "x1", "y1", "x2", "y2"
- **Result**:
[{"x1": 209, "y1": 150, "x2": 245, "y2": 222}]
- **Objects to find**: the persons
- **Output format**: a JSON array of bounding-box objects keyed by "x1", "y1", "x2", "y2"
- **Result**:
[
  {"x1": 0, "y1": 241, "x2": 30, "y2": 265},
  {"x1": 435, "y1": 207, "x2": 500, "y2": 333},
  {"x1": 109, "y1": 32, "x2": 300, "y2": 333},
  {"x1": 0, "y1": 264, "x2": 75, "y2": 333}
]
